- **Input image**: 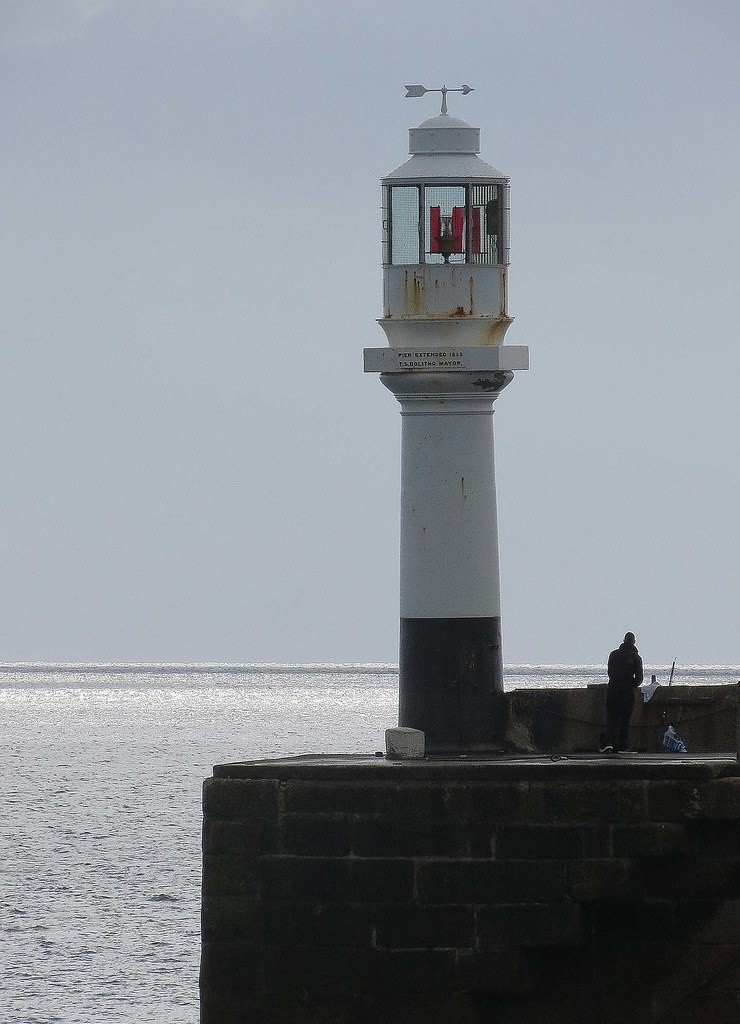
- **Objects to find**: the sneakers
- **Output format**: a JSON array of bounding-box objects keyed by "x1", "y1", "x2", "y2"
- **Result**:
[
  {"x1": 617, "y1": 748, "x2": 639, "y2": 755},
  {"x1": 598, "y1": 743, "x2": 614, "y2": 753}
]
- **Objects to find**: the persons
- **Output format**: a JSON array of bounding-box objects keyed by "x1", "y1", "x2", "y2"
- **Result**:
[{"x1": 599, "y1": 632, "x2": 643, "y2": 754}]
